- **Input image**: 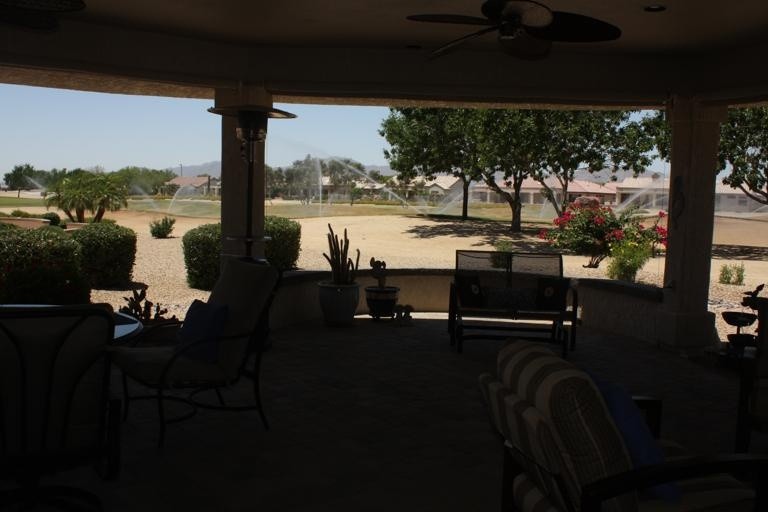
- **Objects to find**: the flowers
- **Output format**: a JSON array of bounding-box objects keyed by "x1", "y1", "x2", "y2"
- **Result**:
[{"x1": 536, "y1": 201, "x2": 668, "y2": 252}]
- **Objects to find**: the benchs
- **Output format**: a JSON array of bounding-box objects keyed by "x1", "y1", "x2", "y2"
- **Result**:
[{"x1": 446, "y1": 249, "x2": 580, "y2": 352}]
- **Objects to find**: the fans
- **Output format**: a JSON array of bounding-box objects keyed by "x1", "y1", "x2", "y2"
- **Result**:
[{"x1": 405, "y1": 0, "x2": 624, "y2": 58}]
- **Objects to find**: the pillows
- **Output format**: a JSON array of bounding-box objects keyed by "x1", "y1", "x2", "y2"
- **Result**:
[
  {"x1": 458, "y1": 275, "x2": 571, "y2": 311},
  {"x1": 583, "y1": 366, "x2": 684, "y2": 499}
]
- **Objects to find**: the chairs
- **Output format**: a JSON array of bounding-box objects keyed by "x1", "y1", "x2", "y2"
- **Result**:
[
  {"x1": 0, "y1": 300, "x2": 122, "y2": 511},
  {"x1": 479, "y1": 336, "x2": 766, "y2": 509},
  {"x1": 112, "y1": 255, "x2": 282, "y2": 448}
]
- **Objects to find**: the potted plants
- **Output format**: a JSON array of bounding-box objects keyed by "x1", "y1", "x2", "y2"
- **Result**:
[
  {"x1": 604, "y1": 229, "x2": 659, "y2": 282},
  {"x1": 317, "y1": 221, "x2": 401, "y2": 328}
]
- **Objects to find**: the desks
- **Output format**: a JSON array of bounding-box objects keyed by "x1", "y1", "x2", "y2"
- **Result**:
[{"x1": 0, "y1": 303, "x2": 144, "y2": 421}]
